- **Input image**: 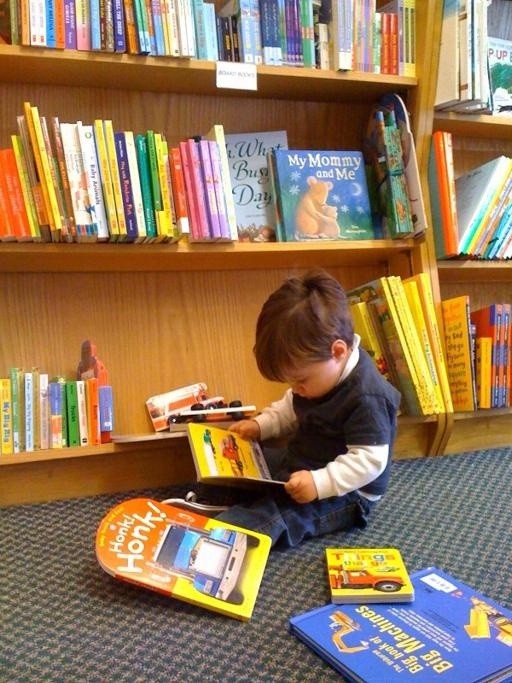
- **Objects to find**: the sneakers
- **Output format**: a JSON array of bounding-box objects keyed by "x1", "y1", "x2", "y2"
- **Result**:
[{"x1": 160, "y1": 488, "x2": 234, "y2": 518}]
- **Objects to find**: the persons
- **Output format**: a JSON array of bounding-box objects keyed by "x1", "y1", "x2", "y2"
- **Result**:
[{"x1": 208, "y1": 265, "x2": 404, "y2": 552}]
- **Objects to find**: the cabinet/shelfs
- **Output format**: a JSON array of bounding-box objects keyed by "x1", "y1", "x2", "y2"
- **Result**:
[
  {"x1": 424, "y1": 1, "x2": 512, "y2": 456},
  {"x1": 1, "y1": 0, "x2": 442, "y2": 508}
]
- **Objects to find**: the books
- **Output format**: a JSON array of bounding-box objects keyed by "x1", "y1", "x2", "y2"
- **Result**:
[
  {"x1": 94, "y1": 496, "x2": 274, "y2": 625},
  {"x1": 429, "y1": 131, "x2": 512, "y2": 261},
  {"x1": 285, "y1": 565, "x2": 511, "y2": 683},
  {"x1": 433, "y1": 0, "x2": 512, "y2": 117},
  {"x1": 1, "y1": 338, "x2": 114, "y2": 455},
  {"x1": 186, "y1": 421, "x2": 289, "y2": 491},
  {"x1": 0, "y1": 101, "x2": 428, "y2": 243},
  {"x1": 324, "y1": 546, "x2": 415, "y2": 605},
  {"x1": 343, "y1": 270, "x2": 512, "y2": 419},
  {"x1": 0, "y1": 0, "x2": 416, "y2": 79}
]
